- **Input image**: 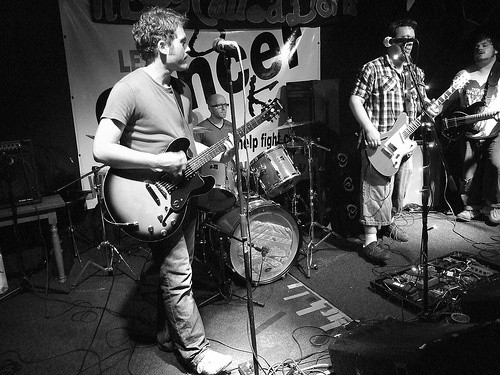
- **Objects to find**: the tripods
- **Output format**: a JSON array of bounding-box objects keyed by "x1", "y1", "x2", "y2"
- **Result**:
[
  {"x1": 0, "y1": 161, "x2": 66, "y2": 301},
  {"x1": 55, "y1": 164, "x2": 138, "y2": 285},
  {"x1": 293, "y1": 137, "x2": 345, "y2": 277},
  {"x1": 59, "y1": 202, "x2": 95, "y2": 268},
  {"x1": 195, "y1": 223, "x2": 264, "y2": 308}
]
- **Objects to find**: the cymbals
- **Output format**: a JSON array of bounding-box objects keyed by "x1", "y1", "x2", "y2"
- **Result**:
[{"x1": 274, "y1": 120, "x2": 313, "y2": 130}]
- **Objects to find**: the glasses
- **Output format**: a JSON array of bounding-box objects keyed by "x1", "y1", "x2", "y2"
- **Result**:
[{"x1": 209, "y1": 103, "x2": 229, "y2": 108}]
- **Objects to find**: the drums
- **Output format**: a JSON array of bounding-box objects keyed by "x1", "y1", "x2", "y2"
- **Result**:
[
  {"x1": 206, "y1": 190, "x2": 303, "y2": 285},
  {"x1": 250, "y1": 144, "x2": 302, "y2": 200},
  {"x1": 195, "y1": 161, "x2": 238, "y2": 214}
]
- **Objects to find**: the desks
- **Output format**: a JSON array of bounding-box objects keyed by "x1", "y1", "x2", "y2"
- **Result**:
[{"x1": 0, "y1": 194, "x2": 67, "y2": 285}]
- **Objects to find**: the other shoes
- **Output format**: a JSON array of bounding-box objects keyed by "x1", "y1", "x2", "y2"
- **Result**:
[
  {"x1": 489, "y1": 207, "x2": 500, "y2": 224},
  {"x1": 190, "y1": 349, "x2": 232, "y2": 375},
  {"x1": 457, "y1": 209, "x2": 481, "y2": 221},
  {"x1": 382, "y1": 222, "x2": 409, "y2": 242},
  {"x1": 363, "y1": 241, "x2": 391, "y2": 260}
]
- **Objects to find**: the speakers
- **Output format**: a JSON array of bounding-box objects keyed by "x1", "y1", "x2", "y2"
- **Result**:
[{"x1": 328, "y1": 319, "x2": 500, "y2": 375}]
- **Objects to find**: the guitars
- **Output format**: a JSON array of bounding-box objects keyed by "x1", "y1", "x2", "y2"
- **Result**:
[
  {"x1": 365, "y1": 72, "x2": 471, "y2": 177},
  {"x1": 99, "y1": 96, "x2": 285, "y2": 242},
  {"x1": 435, "y1": 101, "x2": 500, "y2": 145}
]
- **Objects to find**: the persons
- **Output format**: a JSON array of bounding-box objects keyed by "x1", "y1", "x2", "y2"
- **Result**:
[
  {"x1": 444, "y1": 30, "x2": 500, "y2": 223},
  {"x1": 191, "y1": 95, "x2": 239, "y2": 204},
  {"x1": 349, "y1": 19, "x2": 444, "y2": 262},
  {"x1": 93, "y1": 7, "x2": 242, "y2": 375}
]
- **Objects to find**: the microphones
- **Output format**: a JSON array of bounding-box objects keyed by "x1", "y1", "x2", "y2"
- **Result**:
[
  {"x1": 213, "y1": 37, "x2": 240, "y2": 52},
  {"x1": 2, "y1": 152, "x2": 15, "y2": 165},
  {"x1": 383, "y1": 35, "x2": 418, "y2": 47}
]
